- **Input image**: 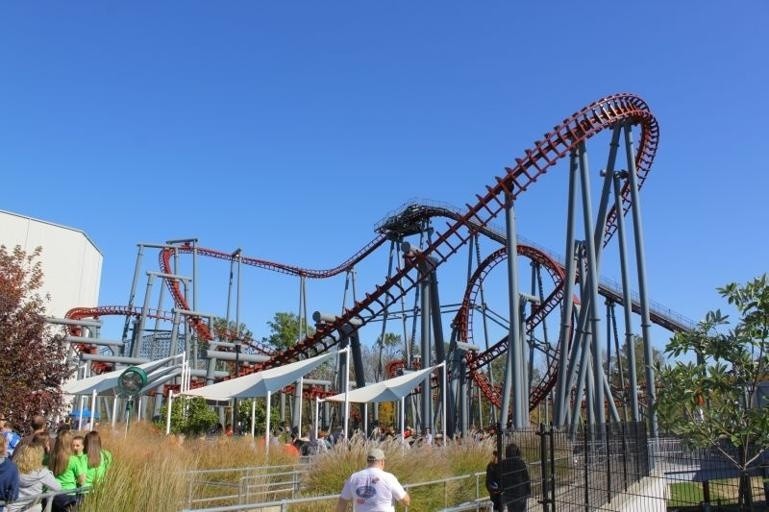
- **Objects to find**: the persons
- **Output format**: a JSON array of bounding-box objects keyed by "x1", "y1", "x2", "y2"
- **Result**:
[{"x1": 688, "y1": 392, "x2": 704, "y2": 445}]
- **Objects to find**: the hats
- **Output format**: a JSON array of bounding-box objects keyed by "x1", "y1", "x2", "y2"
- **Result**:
[{"x1": 366, "y1": 449, "x2": 388, "y2": 461}]
